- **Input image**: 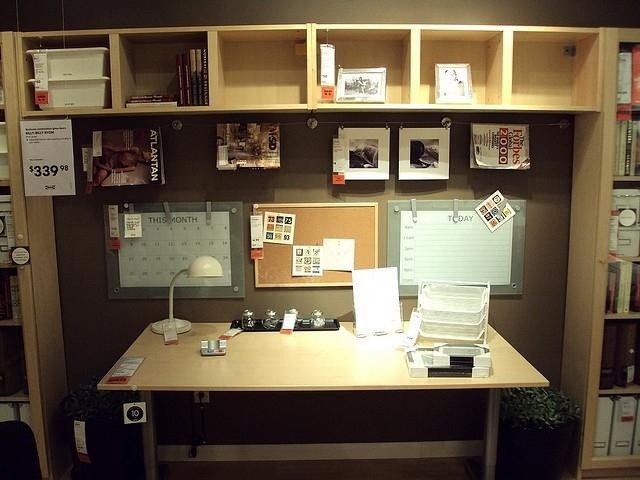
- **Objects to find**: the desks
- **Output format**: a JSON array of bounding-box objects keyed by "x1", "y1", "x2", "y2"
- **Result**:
[{"x1": 98, "y1": 322, "x2": 550, "y2": 480}]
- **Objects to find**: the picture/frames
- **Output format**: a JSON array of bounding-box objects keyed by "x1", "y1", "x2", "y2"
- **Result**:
[
  {"x1": 335, "y1": 67, "x2": 387, "y2": 103},
  {"x1": 435, "y1": 63, "x2": 473, "y2": 104}
]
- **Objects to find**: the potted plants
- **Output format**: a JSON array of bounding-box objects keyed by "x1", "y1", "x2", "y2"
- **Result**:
[
  {"x1": 495, "y1": 387, "x2": 584, "y2": 480},
  {"x1": 57, "y1": 379, "x2": 143, "y2": 480}
]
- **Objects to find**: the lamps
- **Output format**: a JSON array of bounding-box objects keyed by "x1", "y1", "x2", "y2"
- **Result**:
[{"x1": 151, "y1": 255, "x2": 224, "y2": 335}]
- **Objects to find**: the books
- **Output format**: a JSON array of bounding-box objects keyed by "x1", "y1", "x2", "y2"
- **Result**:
[
  {"x1": 591, "y1": 41, "x2": 640, "y2": 459},
  {"x1": 125, "y1": 48, "x2": 209, "y2": 106}
]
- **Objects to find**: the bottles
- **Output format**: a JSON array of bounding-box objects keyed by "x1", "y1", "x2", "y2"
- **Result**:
[{"x1": 242, "y1": 309, "x2": 327, "y2": 329}]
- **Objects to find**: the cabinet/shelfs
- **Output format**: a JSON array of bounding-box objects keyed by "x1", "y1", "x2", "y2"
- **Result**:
[
  {"x1": 0, "y1": 31, "x2": 50, "y2": 480},
  {"x1": 13, "y1": 24, "x2": 605, "y2": 119},
  {"x1": 561, "y1": 27, "x2": 640, "y2": 480}
]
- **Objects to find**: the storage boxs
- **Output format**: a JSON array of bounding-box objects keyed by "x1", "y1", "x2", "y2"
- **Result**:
[
  {"x1": 25, "y1": 47, "x2": 109, "y2": 79},
  {"x1": 27, "y1": 76, "x2": 110, "y2": 111}
]
